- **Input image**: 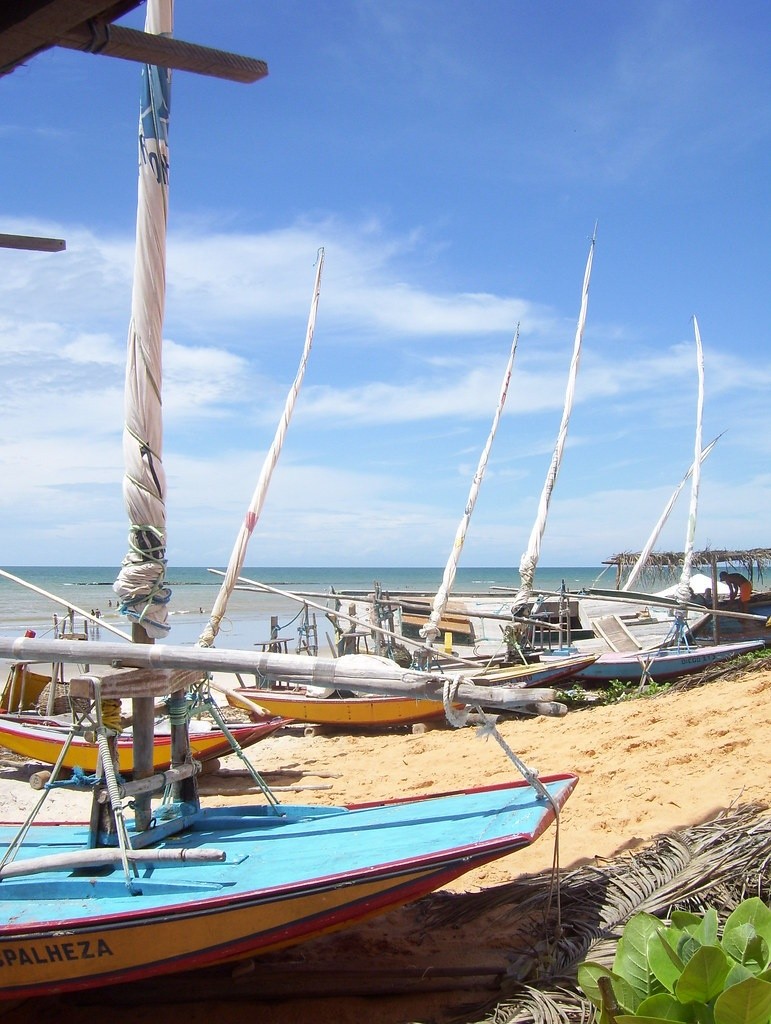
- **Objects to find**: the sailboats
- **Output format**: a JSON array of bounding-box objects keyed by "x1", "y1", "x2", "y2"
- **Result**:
[
  {"x1": 0, "y1": 0, "x2": 580, "y2": 1000},
  {"x1": 0, "y1": 247, "x2": 325, "y2": 780},
  {"x1": 207, "y1": 228, "x2": 767, "y2": 724}
]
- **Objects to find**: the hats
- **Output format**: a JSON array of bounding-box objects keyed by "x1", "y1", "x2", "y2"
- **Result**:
[{"x1": 719, "y1": 571, "x2": 728, "y2": 582}]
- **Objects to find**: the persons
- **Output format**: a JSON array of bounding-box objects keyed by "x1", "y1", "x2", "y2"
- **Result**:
[
  {"x1": 96, "y1": 608, "x2": 101, "y2": 619},
  {"x1": 720, "y1": 572, "x2": 752, "y2": 622},
  {"x1": 90, "y1": 610, "x2": 95, "y2": 624},
  {"x1": 704, "y1": 588, "x2": 712, "y2": 602},
  {"x1": 689, "y1": 587, "x2": 710, "y2": 607}
]
